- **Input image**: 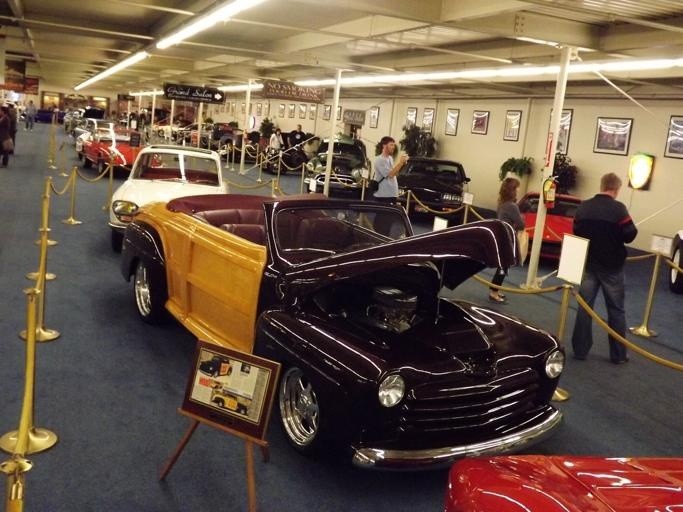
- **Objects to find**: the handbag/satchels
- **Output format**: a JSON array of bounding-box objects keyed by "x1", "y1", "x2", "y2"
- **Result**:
[
  {"x1": 367, "y1": 179, "x2": 379, "y2": 192},
  {"x1": 2, "y1": 138, "x2": 14, "y2": 151},
  {"x1": 516, "y1": 229, "x2": 530, "y2": 267}
]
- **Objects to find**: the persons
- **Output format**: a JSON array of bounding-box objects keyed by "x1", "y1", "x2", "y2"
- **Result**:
[
  {"x1": 488, "y1": 176, "x2": 525, "y2": 304},
  {"x1": 571, "y1": 172, "x2": 637, "y2": 364},
  {"x1": 109, "y1": 101, "x2": 257, "y2": 162},
  {"x1": 22, "y1": 101, "x2": 36, "y2": 131},
  {"x1": 365, "y1": 137, "x2": 408, "y2": 238},
  {"x1": 6, "y1": 103, "x2": 19, "y2": 155},
  {"x1": 0, "y1": 107, "x2": 13, "y2": 169},
  {"x1": 265, "y1": 128, "x2": 285, "y2": 154},
  {"x1": 284, "y1": 123, "x2": 305, "y2": 154}
]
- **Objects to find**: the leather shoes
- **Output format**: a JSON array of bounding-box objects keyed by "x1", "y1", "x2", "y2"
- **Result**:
[
  {"x1": 613, "y1": 353, "x2": 630, "y2": 365},
  {"x1": 488, "y1": 294, "x2": 509, "y2": 304}
]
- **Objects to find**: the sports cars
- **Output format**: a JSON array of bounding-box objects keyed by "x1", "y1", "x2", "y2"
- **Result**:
[
  {"x1": 119, "y1": 194, "x2": 563, "y2": 480},
  {"x1": 515, "y1": 190, "x2": 585, "y2": 262}
]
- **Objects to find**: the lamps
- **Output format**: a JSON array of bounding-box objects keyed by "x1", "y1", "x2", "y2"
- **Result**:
[
  {"x1": 626, "y1": 152, "x2": 655, "y2": 190},
  {"x1": 148, "y1": 0, "x2": 272, "y2": 54},
  {"x1": 71, "y1": 45, "x2": 152, "y2": 92}
]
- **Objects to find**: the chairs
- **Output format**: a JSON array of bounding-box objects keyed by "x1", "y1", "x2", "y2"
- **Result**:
[
  {"x1": 220, "y1": 221, "x2": 269, "y2": 249},
  {"x1": 289, "y1": 216, "x2": 353, "y2": 263}
]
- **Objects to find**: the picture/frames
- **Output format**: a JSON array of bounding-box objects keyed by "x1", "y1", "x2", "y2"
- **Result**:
[
  {"x1": 214, "y1": 104, "x2": 230, "y2": 113},
  {"x1": 405, "y1": 106, "x2": 417, "y2": 130},
  {"x1": 322, "y1": 105, "x2": 331, "y2": 120},
  {"x1": 241, "y1": 104, "x2": 252, "y2": 114},
  {"x1": 420, "y1": 107, "x2": 435, "y2": 134},
  {"x1": 369, "y1": 106, "x2": 379, "y2": 128},
  {"x1": 336, "y1": 106, "x2": 342, "y2": 120},
  {"x1": 278, "y1": 104, "x2": 285, "y2": 117},
  {"x1": 591, "y1": 116, "x2": 634, "y2": 156},
  {"x1": 663, "y1": 115, "x2": 682, "y2": 160},
  {"x1": 309, "y1": 105, "x2": 318, "y2": 120},
  {"x1": 230, "y1": 104, "x2": 236, "y2": 116},
  {"x1": 264, "y1": 104, "x2": 270, "y2": 115},
  {"x1": 542, "y1": 108, "x2": 572, "y2": 157},
  {"x1": 444, "y1": 108, "x2": 460, "y2": 136},
  {"x1": 288, "y1": 104, "x2": 295, "y2": 118},
  {"x1": 503, "y1": 109, "x2": 522, "y2": 142},
  {"x1": 256, "y1": 104, "x2": 262, "y2": 116},
  {"x1": 471, "y1": 110, "x2": 490, "y2": 135},
  {"x1": 299, "y1": 104, "x2": 307, "y2": 119}
]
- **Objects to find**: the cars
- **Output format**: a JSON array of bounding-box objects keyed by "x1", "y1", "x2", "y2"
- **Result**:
[
  {"x1": 443, "y1": 454, "x2": 683, "y2": 512},
  {"x1": 303, "y1": 139, "x2": 371, "y2": 200},
  {"x1": 188, "y1": 347, "x2": 272, "y2": 426},
  {"x1": 33, "y1": 107, "x2": 65, "y2": 124},
  {"x1": 667, "y1": 229, "x2": 681, "y2": 292},
  {"x1": 63, "y1": 106, "x2": 324, "y2": 177},
  {"x1": 388, "y1": 153, "x2": 471, "y2": 229},
  {"x1": 107, "y1": 143, "x2": 228, "y2": 256}
]
